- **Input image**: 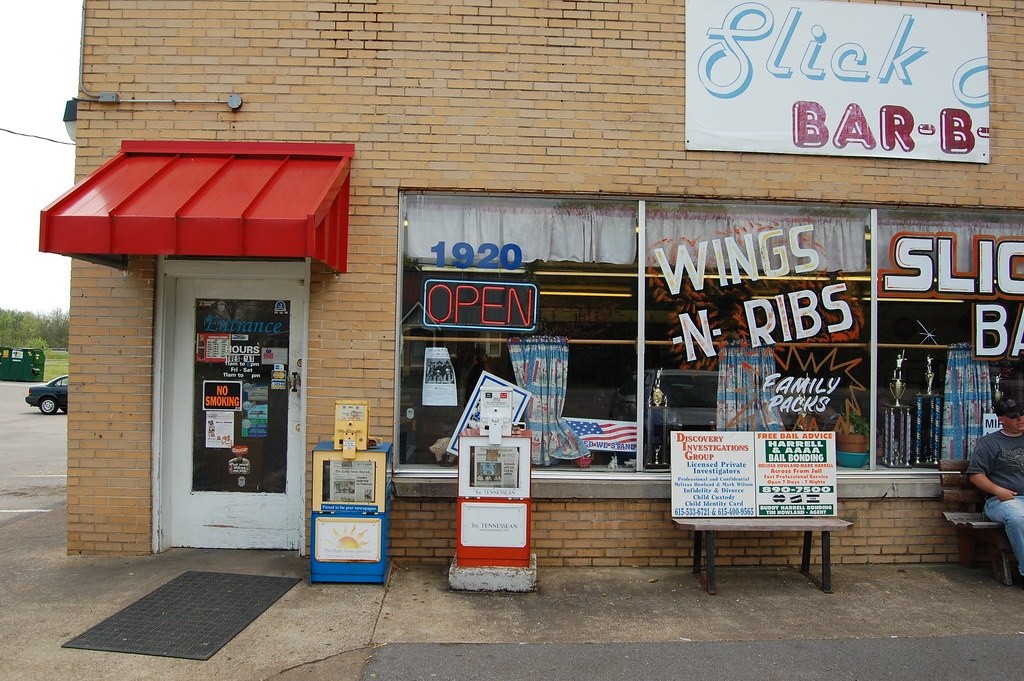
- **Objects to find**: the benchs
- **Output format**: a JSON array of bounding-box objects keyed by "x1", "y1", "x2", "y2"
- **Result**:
[
  {"x1": 672, "y1": 517, "x2": 854, "y2": 595},
  {"x1": 939, "y1": 459, "x2": 1019, "y2": 585}
]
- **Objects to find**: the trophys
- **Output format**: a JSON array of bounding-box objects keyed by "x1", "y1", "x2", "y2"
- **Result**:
[
  {"x1": 913, "y1": 353, "x2": 943, "y2": 468},
  {"x1": 880, "y1": 349, "x2": 912, "y2": 469},
  {"x1": 644, "y1": 366, "x2": 671, "y2": 469},
  {"x1": 991, "y1": 373, "x2": 1005, "y2": 414}
]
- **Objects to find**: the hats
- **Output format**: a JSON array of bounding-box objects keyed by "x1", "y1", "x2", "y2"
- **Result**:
[{"x1": 993, "y1": 397, "x2": 1024, "y2": 413}]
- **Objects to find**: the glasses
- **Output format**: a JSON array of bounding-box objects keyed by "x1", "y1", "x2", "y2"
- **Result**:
[{"x1": 1003, "y1": 410, "x2": 1024, "y2": 419}]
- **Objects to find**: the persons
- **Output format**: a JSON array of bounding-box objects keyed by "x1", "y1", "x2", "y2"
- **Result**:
[
  {"x1": 463, "y1": 346, "x2": 494, "y2": 410},
  {"x1": 426, "y1": 359, "x2": 453, "y2": 384},
  {"x1": 964, "y1": 396, "x2": 1024, "y2": 587}
]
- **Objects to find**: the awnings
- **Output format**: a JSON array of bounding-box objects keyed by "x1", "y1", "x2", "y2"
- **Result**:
[{"x1": 37, "y1": 137, "x2": 355, "y2": 273}]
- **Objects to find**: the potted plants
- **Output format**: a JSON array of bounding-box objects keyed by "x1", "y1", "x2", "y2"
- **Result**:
[{"x1": 836, "y1": 412, "x2": 870, "y2": 452}]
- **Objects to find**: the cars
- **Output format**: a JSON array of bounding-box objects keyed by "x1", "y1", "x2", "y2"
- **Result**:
[
  {"x1": 611, "y1": 368, "x2": 779, "y2": 468},
  {"x1": 25, "y1": 374, "x2": 68, "y2": 415}
]
